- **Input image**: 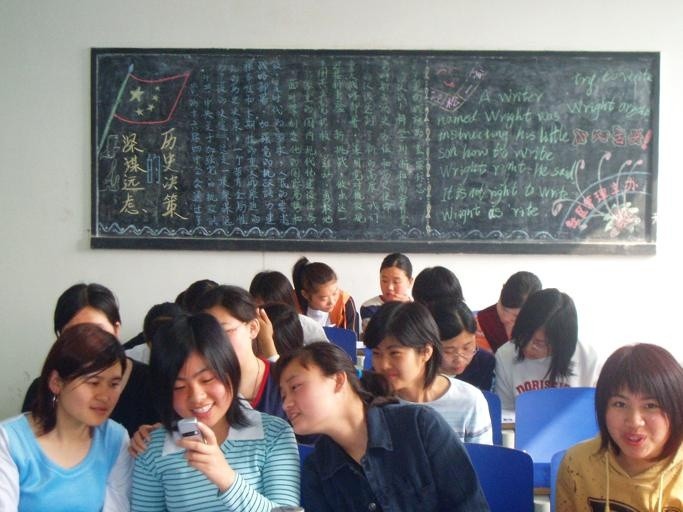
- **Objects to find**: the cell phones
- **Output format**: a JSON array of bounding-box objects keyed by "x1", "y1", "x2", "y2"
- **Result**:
[{"x1": 177, "y1": 417, "x2": 204, "y2": 444}]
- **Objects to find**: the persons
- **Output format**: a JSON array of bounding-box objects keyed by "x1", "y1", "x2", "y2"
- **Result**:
[
  {"x1": 0, "y1": 323, "x2": 133, "y2": 512},
  {"x1": 21, "y1": 254, "x2": 594, "y2": 446},
  {"x1": 125, "y1": 340, "x2": 491, "y2": 511},
  {"x1": 553, "y1": 344, "x2": 683, "y2": 511},
  {"x1": 126, "y1": 309, "x2": 301, "y2": 511}
]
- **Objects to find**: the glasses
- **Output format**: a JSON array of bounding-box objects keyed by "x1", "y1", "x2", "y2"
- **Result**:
[{"x1": 440, "y1": 340, "x2": 479, "y2": 362}]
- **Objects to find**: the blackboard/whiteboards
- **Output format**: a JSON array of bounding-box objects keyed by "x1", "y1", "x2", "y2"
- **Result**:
[{"x1": 90, "y1": 46, "x2": 660, "y2": 254}]
